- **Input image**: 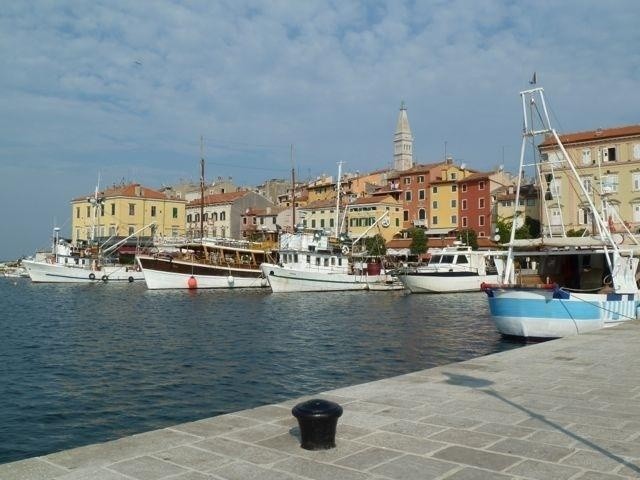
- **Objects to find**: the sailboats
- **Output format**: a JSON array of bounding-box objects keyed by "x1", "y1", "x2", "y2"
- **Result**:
[{"x1": 134, "y1": 134, "x2": 320, "y2": 299}]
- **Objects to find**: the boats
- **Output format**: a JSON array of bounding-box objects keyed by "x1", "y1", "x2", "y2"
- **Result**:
[
  {"x1": 0, "y1": 261, "x2": 28, "y2": 280},
  {"x1": 22, "y1": 169, "x2": 162, "y2": 286},
  {"x1": 398, "y1": 238, "x2": 518, "y2": 295},
  {"x1": 257, "y1": 153, "x2": 405, "y2": 295},
  {"x1": 366, "y1": 280, "x2": 405, "y2": 292},
  {"x1": 480, "y1": 68, "x2": 640, "y2": 342}
]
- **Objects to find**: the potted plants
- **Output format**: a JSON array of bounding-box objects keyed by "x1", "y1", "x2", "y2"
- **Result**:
[{"x1": 365, "y1": 233, "x2": 388, "y2": 276}]
